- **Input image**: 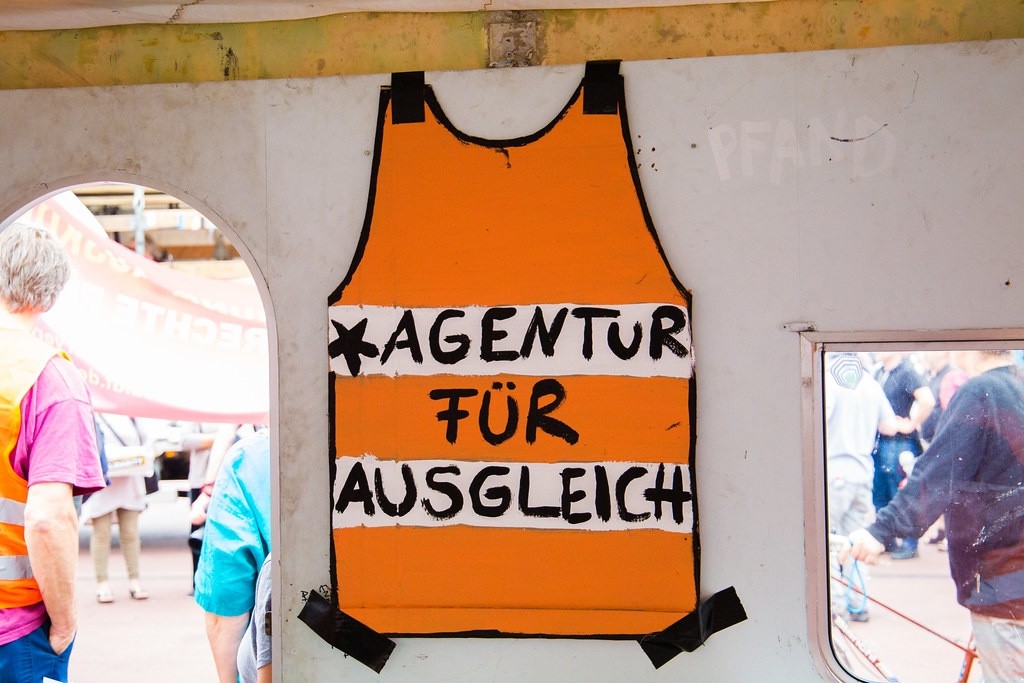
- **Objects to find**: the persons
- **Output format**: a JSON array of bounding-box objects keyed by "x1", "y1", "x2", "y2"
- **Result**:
[
  {"x1": 826, "y1": 351, "x2": 1024, "y2": 683},
  {"x1": 0, "y1": 219, "x2": 277, "y2": 683}
]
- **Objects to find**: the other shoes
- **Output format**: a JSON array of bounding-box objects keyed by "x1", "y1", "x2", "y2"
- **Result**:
[
  {"x1": 847, "y1": 600, "x2": 868, "y2": 621},
  {"x1": 885, "y1": 538, "x2": 897, "y2": 552},
  {"x1": 96, "y1": 583, "x2": 113, "y2": 603},
  {"x1": 130, "y1": 579, "x2": 149, "y2": 598},
  {"x1": 891, "y1": 543, "x2": 919, "y2": 560},
  {"x1": 928, "y1": 530, "x2": 946, "y2": 543},
  {"x1": 938, "y1": 537, "x2": 948, "y2": 551}
]
